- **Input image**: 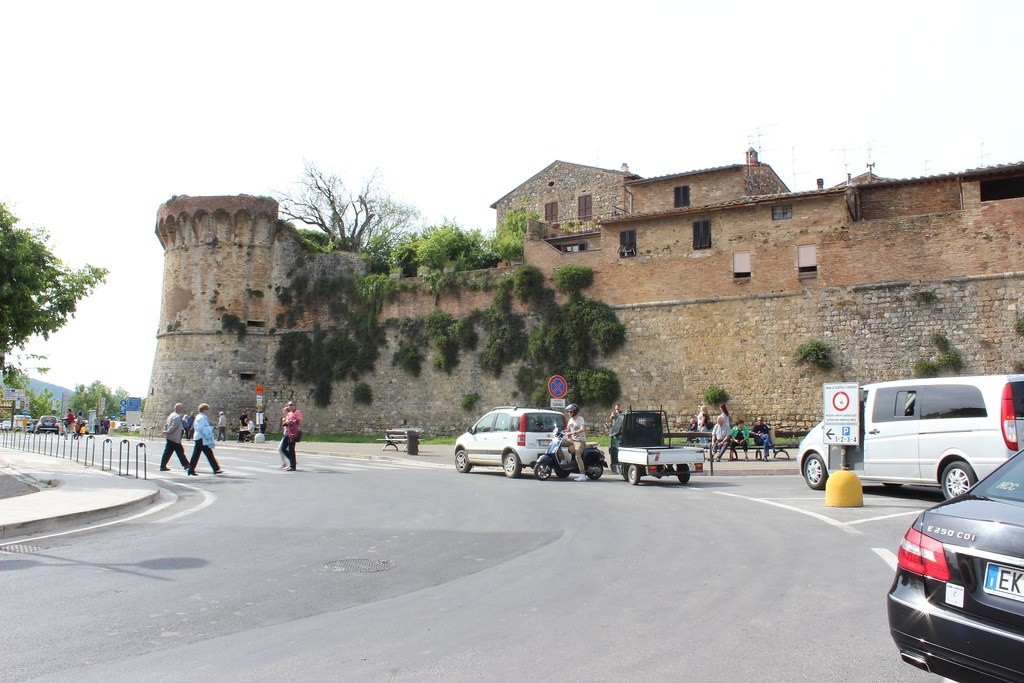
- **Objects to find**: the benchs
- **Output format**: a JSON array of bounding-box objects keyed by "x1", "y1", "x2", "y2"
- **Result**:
[
  {"x1": 376, "y1": 429, "x2": 425, "y2": 453},
  {"x1": 702, "y1": 430, "x2": 810, "y2": 462}
]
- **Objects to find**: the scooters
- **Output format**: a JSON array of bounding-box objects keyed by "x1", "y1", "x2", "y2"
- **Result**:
[{"x1": 533, "y1": 423, "x2": 608, "y2": 481}]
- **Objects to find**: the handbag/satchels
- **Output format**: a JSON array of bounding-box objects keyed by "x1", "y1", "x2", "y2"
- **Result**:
[
  {"x1": 295, "y1": 430, "x2": 302, "y2": 442},
  {"x1": 705, "y1": 421, "x2": 715, "y2": 430}
]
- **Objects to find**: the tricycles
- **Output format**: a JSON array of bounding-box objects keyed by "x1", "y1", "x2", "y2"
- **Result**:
[{"x1": 608, "y1": 405, "x2": 705, "y2": 485}]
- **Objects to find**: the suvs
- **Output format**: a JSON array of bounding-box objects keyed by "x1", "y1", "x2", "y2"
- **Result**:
[
  {"x1": 454, "y1": 406, "x2": 568, "y2": 479},
  {"x1": 34, "y1": 415, "x2": 59, "y2": 434}
]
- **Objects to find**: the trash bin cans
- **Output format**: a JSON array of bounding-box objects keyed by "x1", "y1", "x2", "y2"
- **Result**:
[{"x1": 407, "y1": 431, "x2": 418, "y2": 455}]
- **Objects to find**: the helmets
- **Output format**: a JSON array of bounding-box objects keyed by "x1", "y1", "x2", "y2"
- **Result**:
[{"x1": 565, "y1": 404, "x2": 580, "y2": 414}]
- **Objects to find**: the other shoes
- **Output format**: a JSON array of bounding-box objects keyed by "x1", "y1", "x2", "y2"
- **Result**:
[
  {"x1": 574, "y1": 474, "x2": 587, "y2": 482},
  {"x1": 160, "y1": 464, "x2": 223, "y2": 476},
  {"x1": 730, "y1": 457, "x2": 748, "y2": 462},
  {"x1": 279, "y1": 464, "x2": 296, "y2": 471},
  {"x1": 708, "y1": 455, "x2": 720, "y2": 462},
  {"x1": 559, "y1": 459, "x2": 568, "y2": 465},
  {"x1": 763, "y1": 456, "x2": 768, "y2": 461},
  {"x1": 769, "y1": 443, "x2": 773, "y2": 449}
]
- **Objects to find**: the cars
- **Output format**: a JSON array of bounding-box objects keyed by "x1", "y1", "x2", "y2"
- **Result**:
[
  {"x1": 128, "y1": 424, "x2": 141, "y2": 432},
  {"x1": 2, "y1": 421, "x2": 11, "y2": 431},
  {"x1": 886, "y1": 448, "x2": 1024, "y2": 683},
  {"x1": 25, "y1": 422, "x2": 34, "y2": 433}
]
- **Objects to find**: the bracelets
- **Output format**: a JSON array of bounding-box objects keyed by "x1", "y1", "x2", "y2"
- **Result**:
[{"x1": 573, "y1": 432, "x2": 576, "y2": 436}]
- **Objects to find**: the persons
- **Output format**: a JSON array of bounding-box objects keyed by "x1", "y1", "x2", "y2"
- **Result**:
[
  {"x1": 708, "y1": 415, "x2": 732, "y2": 462},
  {"x1": 188, "y1": 403, "x2": 223, "y2": 476},
  {"x1": 75, "y1": 411, "x2": 90, "y2": 440},
  {"x1": 159, "y1": 403, "x2": 190, "y2": 471},
  {"x1": 94, "y1": 415, "x2": 110, "y2": 435},
  {"x1": 236, "y1": 408, "x2": 254, "y2": 443},
  {"x1": 682, "y1": 404, "x2": 729, "y2": 452},
  {"x1": 610, "y1": 404, "x2": 622, "y2": 426},
  {"x1": 560, "y1": 403, "x2": 586, "y2": 481},
  {"x1": 260, "y1": 414, "x2": 268, "y2": 434},
  {"x1": 181, "y1": 412, "x2": 195, "y2": 441},
  {"x1": 751, "y1": 416, "x2": 774, "y2": 462},
  {"x1": 279, "y1": 400, "x2": 302, "y2": 471},
  {"x1": 731, "y1": 420, "x2": 749, "y2": 462},
  {"x1": 216, "y1": 411, "x2": 227, "y2": 442},
  {"x1": 64, "y1": 408, "x2": 74, "y2": 440}
]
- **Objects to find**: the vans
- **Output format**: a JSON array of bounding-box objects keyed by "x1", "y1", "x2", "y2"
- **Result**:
[{"x1": 796, "y1": 374, "x2": 1024, "y2": 500}]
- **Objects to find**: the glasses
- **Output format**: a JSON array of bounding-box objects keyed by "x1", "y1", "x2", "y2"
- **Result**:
[
  {"x1": 567, "y1": 411, "x2": 573, "y2": 413},
  {"x1": 757, "y1": 420, "x2": 762, "y2": 422}
]
